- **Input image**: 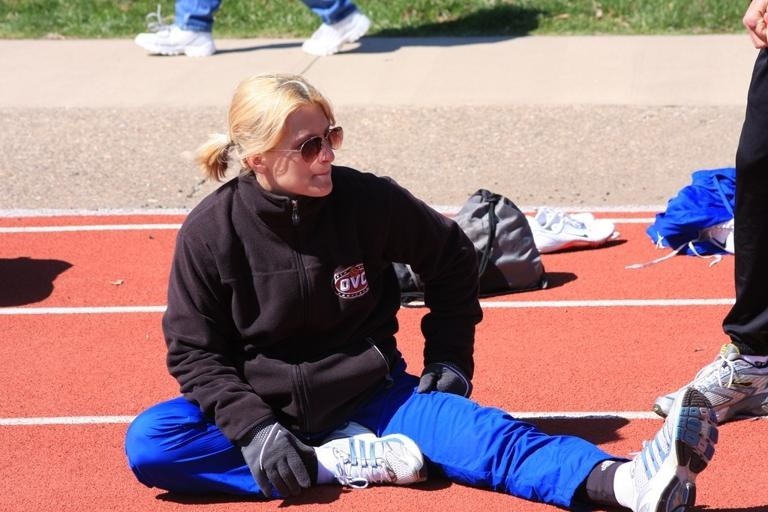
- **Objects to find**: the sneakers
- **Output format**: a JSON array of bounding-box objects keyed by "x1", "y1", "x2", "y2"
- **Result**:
[
  {"x1": 302, "y1": 13, "x2": 374, "y2": 58},
  {"x1": 135, "y1": 4, "x2": 217, "y2": 58},
  {"x1": 524, "y1": 206, "x2": 616, "y2": 253},
  {"x1": 320, "y1": 418, "x2": 430, "y2": 492},
  {"x1": 651, "y1": 344, "x2": 768, "y2": 428},
  {"x1": 629, "y1": 386, "x2": 720, "y2": 512}
]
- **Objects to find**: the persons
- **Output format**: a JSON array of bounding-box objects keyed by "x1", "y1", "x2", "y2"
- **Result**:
[
  {"x1": 134, "y1": 1, "x2": 374, "y2": 58},
  {"x1": 126, "y1": 73, "x2": 719, "y2": 511},
  {"x1": 652, "y1": 1, "x2": 767, "y2": 423}
]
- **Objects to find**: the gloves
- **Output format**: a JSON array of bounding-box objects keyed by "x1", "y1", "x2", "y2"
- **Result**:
[
  {"x1": 236, "y1": 417, "x2": 319, "y2": 500},
  {"x1": 415, "y1": 362, "x2": 468, "y2": 396}
]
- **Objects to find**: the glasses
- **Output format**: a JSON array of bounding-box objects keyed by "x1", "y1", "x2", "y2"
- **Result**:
[{"x1": 266, "y1": 126, "x2": 344, "y2": 164}]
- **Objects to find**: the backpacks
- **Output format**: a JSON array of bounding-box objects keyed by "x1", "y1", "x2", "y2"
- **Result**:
[{"x1": 455, "y1": 186, "x2": 547, "y2": 295}]
